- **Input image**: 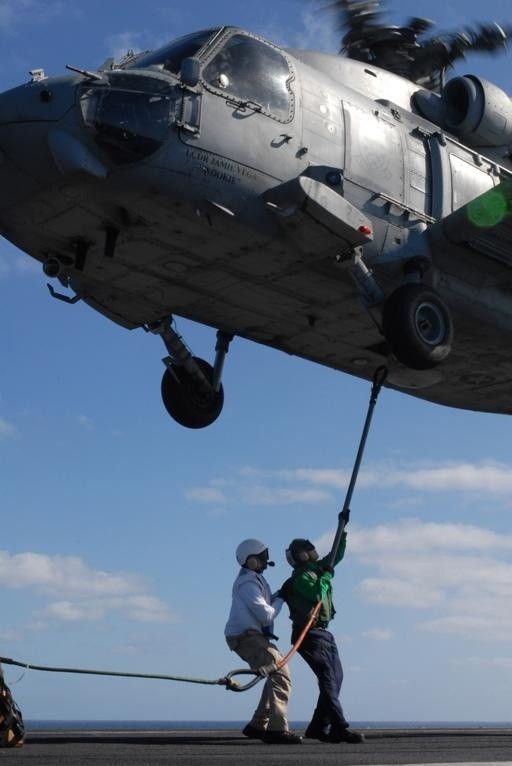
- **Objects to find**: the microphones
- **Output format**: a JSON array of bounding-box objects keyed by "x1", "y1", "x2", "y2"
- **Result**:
[{"x1": 266, "y1": 562, "x2": 275, "y2": 566}]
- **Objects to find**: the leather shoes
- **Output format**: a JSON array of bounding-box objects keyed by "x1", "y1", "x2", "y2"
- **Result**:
[
  {"x1": 242, "y1": 721, "x2": 303, "y2": 744},
  {"x1": 305, "y1": 724, "x2": 365, "y2": 743}
]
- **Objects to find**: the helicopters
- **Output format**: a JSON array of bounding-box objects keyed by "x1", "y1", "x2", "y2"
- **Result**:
[{"x1": 0, "y1": 1, "x2": 512, "y2": 430}]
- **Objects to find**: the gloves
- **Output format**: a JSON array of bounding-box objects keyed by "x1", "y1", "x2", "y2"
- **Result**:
[
  {"x1": 323, "y1": 564, "x2": 335, "y2": 579},
  {"x1": 337, "y1": 508, "x2": 350, "y2": 527}
]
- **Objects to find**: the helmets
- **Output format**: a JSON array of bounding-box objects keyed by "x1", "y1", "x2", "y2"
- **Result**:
[
  {"x1": 286, "y1": 538, "x2": 315, "y2": 569},
  {"x1": 236, "y1": 538, "x2": 269, "y2": 566}
]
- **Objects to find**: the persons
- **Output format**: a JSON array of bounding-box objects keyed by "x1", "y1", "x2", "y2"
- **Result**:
[
  {"x1": 276, "y1": 507, "x2": 367, "y2": 745},
  {"x1": 222, "y1": 536, "x2": 304, "y2": 745}
]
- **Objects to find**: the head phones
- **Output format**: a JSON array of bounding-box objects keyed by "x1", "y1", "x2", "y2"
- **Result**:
[
  {"x1": 292, "y1": 540, "x2": 310, "y2": 562},
  {"x1": 246, "y1": 555, "x2": 262, "y2": 570}
]
